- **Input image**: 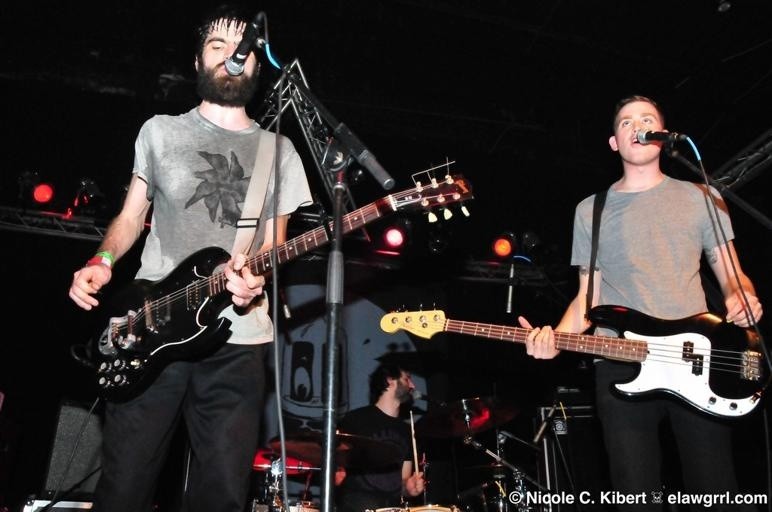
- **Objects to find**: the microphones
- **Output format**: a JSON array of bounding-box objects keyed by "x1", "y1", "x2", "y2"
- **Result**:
[
  {"x1": 636, "y1": 127, "x2": 677, "y2": 146},
  {"x1": 412, "y1": 391, "x2": 444, "y2": 408},
  {"x1": 531, "y1": 404, "x2": 557, "y2": 445},
  {"x1": 224, "y1": 22, "x2": 263, "y2": 76}
]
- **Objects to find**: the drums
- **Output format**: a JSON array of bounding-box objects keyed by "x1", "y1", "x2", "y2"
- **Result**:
[
  {"x1": 367, "y1": 504, "x2": 461, "y2": 512},
  {"x1": 250, "y1": 496, "x2": 337, "y2": 512}
]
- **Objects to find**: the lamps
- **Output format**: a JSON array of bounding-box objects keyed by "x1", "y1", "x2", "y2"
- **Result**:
[
  {"x1": 492, "y1": 228, "x2": 518, "y2": 258},
  {"x1": 17, "y1": 170, "x2": 56, "y2": 206},
  {"x1": 385, "y1": 214, "x2": 412, "y2": 247}
]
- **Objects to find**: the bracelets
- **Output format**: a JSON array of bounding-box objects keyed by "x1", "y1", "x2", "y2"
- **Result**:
[
  {"x1": 94, "y1": 251, "x2": 114, "y2": 267},
  {"x1": 86, "y1": 256, "x2": 113, "y2": 270}
]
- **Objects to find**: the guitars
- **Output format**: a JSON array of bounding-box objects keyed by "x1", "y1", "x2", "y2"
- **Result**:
[
  {"x1": 71, "y1": 162, "x2": 474, "y2": 402},
  {"x1": 380, "y1": 303, "x2": 771, "y2": 424}
]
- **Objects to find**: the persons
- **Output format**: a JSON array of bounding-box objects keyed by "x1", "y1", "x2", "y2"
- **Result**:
[
  {"x1": 518, "y1": 95, "x2": 763, "y2": 512},
  {"x1": 67, "y1": 2, "x2": 313, "y2": 509},
  {"x1": 326, "y1": 363, "x2": 428, "y2": 511}
]
